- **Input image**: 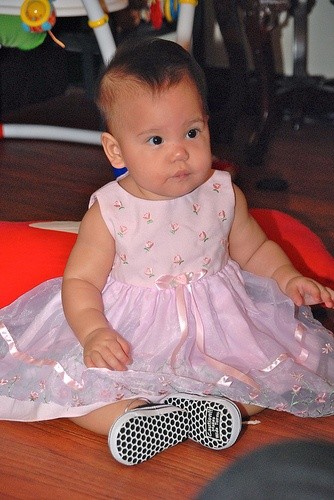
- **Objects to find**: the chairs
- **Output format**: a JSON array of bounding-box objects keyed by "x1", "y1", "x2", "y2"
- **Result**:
[{"x1": 209, "y1": 0, "x2": 322, "y2": 153}]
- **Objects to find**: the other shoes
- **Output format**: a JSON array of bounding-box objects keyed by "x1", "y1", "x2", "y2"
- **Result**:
[
  {"x1": 159, "y1": 392, "x2": 243, "y2": 451},
  {"x1": 108, "y1": 403, "x2": 190, "y2": 465}
]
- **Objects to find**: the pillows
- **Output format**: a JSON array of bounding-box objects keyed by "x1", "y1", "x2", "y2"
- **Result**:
[{"x1": 1, "y1": 209, "x2": 334, "y2": 327}]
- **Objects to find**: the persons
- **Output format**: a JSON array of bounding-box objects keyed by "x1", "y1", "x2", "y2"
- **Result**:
[{"x1": 0, "y1": 38, "x2": 333, "y2": 466}]
